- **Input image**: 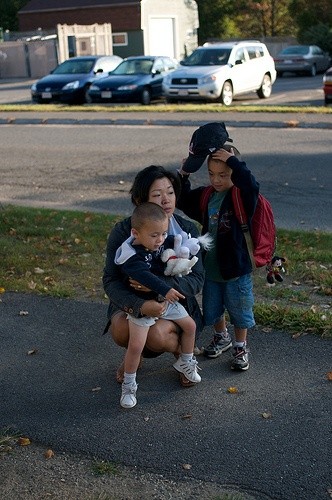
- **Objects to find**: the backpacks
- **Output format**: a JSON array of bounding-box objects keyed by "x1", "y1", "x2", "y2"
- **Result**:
[{"x1": 200, "y1": 185, "x2": 277, "y2": 267}]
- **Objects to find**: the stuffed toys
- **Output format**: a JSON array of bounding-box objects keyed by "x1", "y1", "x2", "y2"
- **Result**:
[
  {"x1": 161, "y1": 234, "x2": 212, "y2": 278},
  {"x1": 267, "y1": 256, "x2": 285, "y2": 284}
]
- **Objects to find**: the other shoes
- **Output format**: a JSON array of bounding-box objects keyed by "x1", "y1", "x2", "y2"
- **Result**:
[
  {"x1": 180, "y1": 373, "x2": 195, "y2": 387},
  {"x1": 116, "y1": 354, "x2": 144, "y2": 383}
]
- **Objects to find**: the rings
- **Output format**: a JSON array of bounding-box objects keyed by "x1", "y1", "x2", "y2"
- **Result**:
[{"x1": 138, "y1": 285, "x2": 142, "y2": 289}]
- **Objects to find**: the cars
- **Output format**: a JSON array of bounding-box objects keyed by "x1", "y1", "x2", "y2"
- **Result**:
[
  {"x1": 274, "y1": 44, "x2": 332, "y2": 77},
  {"x1": 87, "y1": 54, "x2": 179, "y2": 106},
  {"x1": 30, "y1": 54, "x2": 126, "y2": 105}
]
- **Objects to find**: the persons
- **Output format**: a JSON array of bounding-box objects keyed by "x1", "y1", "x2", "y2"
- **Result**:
[
  {"x1": 114, "y1": 203, "x2": 203, "y2": 407},
  {"x1": 103, "y1": 166, "x2": 205, "y2": 386},
  {"x1": 172, "y1": 145, "x2": 259, "y2": 371}
]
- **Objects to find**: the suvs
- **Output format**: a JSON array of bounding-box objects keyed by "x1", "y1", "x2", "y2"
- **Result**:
[{"x1": 161, "y1": 39, "x2": 277, "y2": 106}]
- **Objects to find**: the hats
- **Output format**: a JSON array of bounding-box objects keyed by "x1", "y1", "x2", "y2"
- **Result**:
[{"x1": 182, "y1": 122, "x2": 233, "y2": 173}]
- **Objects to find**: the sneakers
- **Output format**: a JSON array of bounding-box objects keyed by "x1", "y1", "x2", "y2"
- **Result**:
[
  {"x1": 120, "y1": 380, "x2": 137, "y2": 409},
  {"x1": 172, "y1": 357, "x2": 202, "y2": 383},
  {"x1": 230, "y1": 341, "x2": 249, "y2": 370},
  {"x1": 203, "y1": 331, "x2": 233, "y2": 357}
]
